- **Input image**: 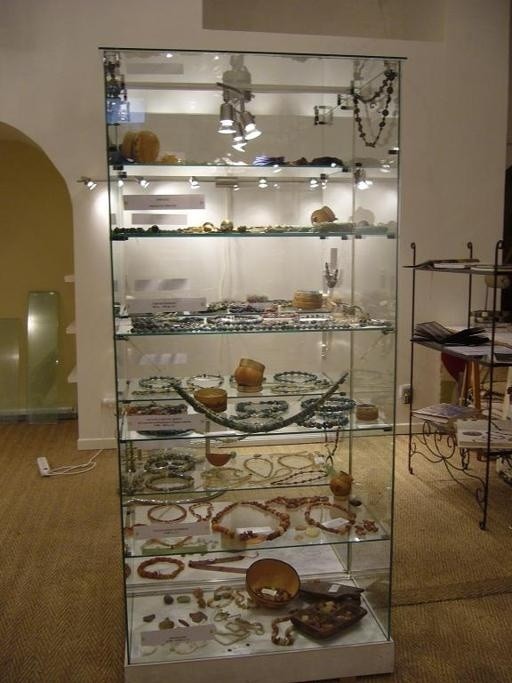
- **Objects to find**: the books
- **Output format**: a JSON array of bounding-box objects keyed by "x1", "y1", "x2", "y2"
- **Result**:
[
  {"x1": 453, "y1": 416, "x2": 511, "y2": 451},
  {"x1": 409, "y1": 399, "x2": 474, "y2": 427},
  {"x1": 470, "y1": 263, "x2": 511, "y2": 273},
  {"x1": 409, "y1": 306, "x2": 512, "y2": 347},
  {"x1": 431, "y1": 260, "x2": 492, "y2": 269}
]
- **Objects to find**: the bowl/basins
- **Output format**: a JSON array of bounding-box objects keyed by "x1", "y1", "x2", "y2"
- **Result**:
[
  {"x1": 244, "y1": 557, "x2": 302, "y2": 609},
  {"x1": 205, "y1": 453, "x2": 231, "y2": 466}
]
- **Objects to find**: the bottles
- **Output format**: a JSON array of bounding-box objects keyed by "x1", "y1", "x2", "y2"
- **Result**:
[
  {"x1": 328, "y1": 470, "x2": 352, "y2": 496},
  {"x1": 235, "y1": 357, "x2": 264, "y2": 386},
  {"x1": 310, "y1": 205, "x2": 337, "y2": 225},
  {"x1": 120, "y1": 129, "x2": 160, "y2": 161}
]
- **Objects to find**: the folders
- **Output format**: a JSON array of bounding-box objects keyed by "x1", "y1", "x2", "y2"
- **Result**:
[{"x1": 403, "y1": 258, "x2": 479, "y2": 268}]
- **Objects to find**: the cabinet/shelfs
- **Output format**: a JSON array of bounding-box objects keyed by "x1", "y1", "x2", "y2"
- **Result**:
[
  {"x1": 97, "y1": 42, "x2": 407, "y2": 682},
  {"x1": 396, "y1": 232, "x2": 512, "y2": 532}
]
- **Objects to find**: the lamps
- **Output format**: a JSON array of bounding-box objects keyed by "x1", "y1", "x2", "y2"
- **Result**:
[
  {"x1": 256, "y1": 176, "x2": 373, "y2": 191},
  {"x1": 76, "y1": 174, "x2": 150, "y2": 190},
  {"x1": 213, "y1": 81, "x2": 264, "y2": 154},
  {"x1": 185, "y1": 174, "x2": 204, "y2": 189}
]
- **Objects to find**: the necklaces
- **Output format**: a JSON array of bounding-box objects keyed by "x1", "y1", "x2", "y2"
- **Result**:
[
  {"x1": 159, "y1": 634, "x2": 200, "y2": 656},
  {"x1": 350, "y1": 69, "x2": 400, "y2": 148},
  {"x1": 271, "y1": 594, "x2": 363, "y2": 647},
  {"x1": 121, "y1": 498, "x2": 357, "y2": 543},
  {"x1": 110, "y1": 223, "x2": 358, "y2": 235},
  {"x1": 116, "y1": 293, "x2": 392, "y2": 335},
  {"x1": 119, "y1": 448, "x2": 330, "y2": 494},
  {"x1": 137, "y1": 558, "x2": 185, "y2": 580},
  {"x1": 127, "y1": 372, "x2": 356, "y2": 465},
  {"x1": 206, "y1": 585, "x2": 264, "y2": 646}
]
